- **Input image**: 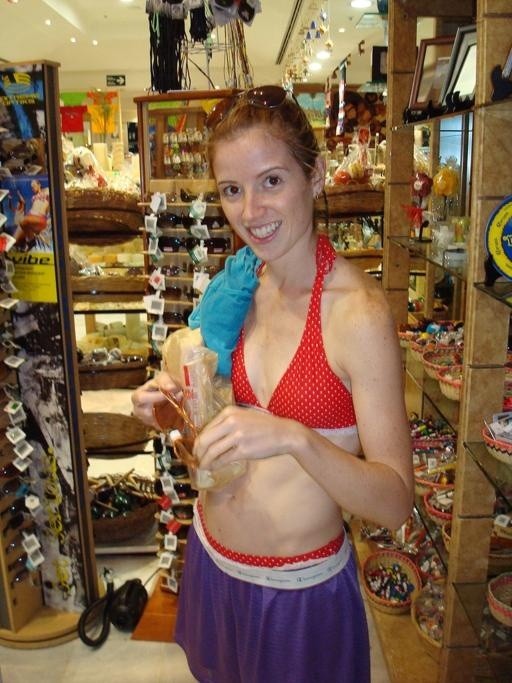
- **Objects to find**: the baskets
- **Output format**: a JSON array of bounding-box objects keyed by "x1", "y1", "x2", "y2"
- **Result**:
[
  {"x1": 78, "y1": 353, "x2": 147, "y2": 390},
  {"x1": 70, "y1": 275, "x2": 150, "y2": 303},
  {"x1": 316, "y1": 181, "x2": 385, "y2": 223},
  {"x1": 398, "y1": 320, "x2": 512, "y2": 402},
  {"x1": 412, "y1": 578, "x2": 511, "y2": 678},
  {"x1": 88, "y1": 475, "x2": 162, "y2": 544},
  {"x1": 482, "y1": 424, "x2": 512, "y2": 466},
  {"x1": 79, "y1": 412, "x2": 154, "y2": 459},
  {"x1": 489, "y1": 572, "x2": 512, "y2": 627},
  {"x1": 64, "y1": 186, "x2": 143, "y2": 247},
  {"x1": 341, "y1": 249, "x2": 383, "y2": 268},
  {"x1": 412, "y1": 463, "x2": 512, "y2": 577},
  {"x1": 361, "y1": 550, "x2": 422, "y2": 616}
]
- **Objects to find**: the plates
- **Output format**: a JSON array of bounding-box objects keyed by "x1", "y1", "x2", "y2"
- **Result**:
[{"x1": 486, "y1": 195, "x2": 512, "y2": 285}]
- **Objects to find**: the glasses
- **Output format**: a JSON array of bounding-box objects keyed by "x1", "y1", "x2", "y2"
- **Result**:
[
  {"x1": 147, "y1": 189, "x2": 232, "y2": 326},
  {"x1": 154, "y1": 484, "x2": 198, "y2": 596},
  {"x1": 0, "y1": 424, "x2": 43, "y2": 590},
  {"x1": 153, "y1": 388, "x2": 244, "y2": 488},
  {"x1": 203, "y1": 85, "x2": 309, "y2": 131}
]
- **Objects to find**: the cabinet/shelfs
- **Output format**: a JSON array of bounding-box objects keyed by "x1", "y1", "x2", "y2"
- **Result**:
[
  {"x1": 3, "y1": 60, "x2": 390, "y2": 646},
  {"x1": 379, "y1": 2, "x2": 508, "y2": 679}
]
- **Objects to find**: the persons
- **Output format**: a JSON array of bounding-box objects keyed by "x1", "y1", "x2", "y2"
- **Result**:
[
  {"x1": 130, "y1": 85, "x2": 416, "y2": 683},
  {"x1": 0, "y1": 119, "x2": 50, "y2": 252}
]
